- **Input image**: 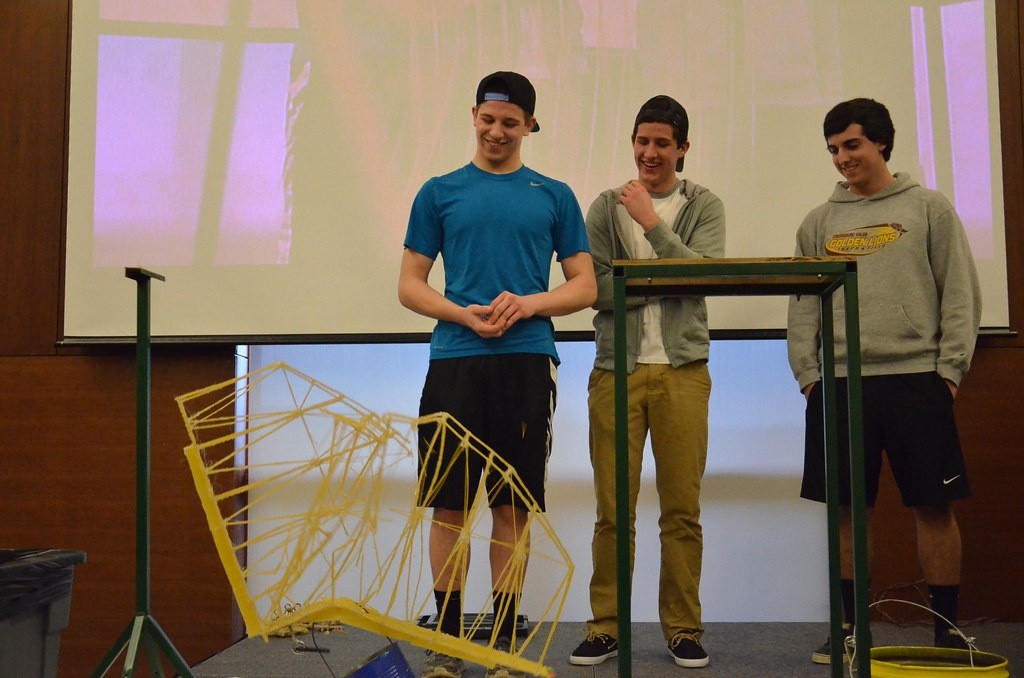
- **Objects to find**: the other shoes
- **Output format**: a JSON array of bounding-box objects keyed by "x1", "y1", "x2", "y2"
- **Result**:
[{"x1": 812, "y1": 616, "x2": 857, "y2": 663}]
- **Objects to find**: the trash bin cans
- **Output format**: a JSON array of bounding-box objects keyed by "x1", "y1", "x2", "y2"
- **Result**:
[{"x1": 1, "y1": 543, "x2": 87, "y2": 678}]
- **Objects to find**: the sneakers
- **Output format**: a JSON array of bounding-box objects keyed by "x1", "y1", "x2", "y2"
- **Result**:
[
  {"x1": 484, "y1": 635, "x2": 526, "y2": 678},
  {"x1": 570, "y1": 631, "x2": 619, "y2": 665},
  {"x1": 420, "y1": 647, "x2": 467, "y2": 678},
  {"x1": 666, "y1": 630, "x2": 709, "y2": 667}
]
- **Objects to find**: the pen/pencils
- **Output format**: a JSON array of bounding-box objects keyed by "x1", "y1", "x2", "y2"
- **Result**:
[{"x1": 481, "y1": 313, "x2": 490, "y2": 322}]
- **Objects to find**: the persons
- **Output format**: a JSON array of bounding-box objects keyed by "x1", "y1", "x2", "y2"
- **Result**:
[
  {"x1": 398, "y1": 70, "x2": 596, "y2": 677},
  {"x1": 570, "y1": 94, "x2": 726, "y2": 668},
  {"x1": 787, "y1": 98, "x2": 984, "y2": 664}
]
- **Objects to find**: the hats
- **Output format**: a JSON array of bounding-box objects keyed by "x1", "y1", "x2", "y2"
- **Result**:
[
  {"x1": 476, "y1": 72, "x2": 540, "y2": 133},
  {"x1": 634, "y1": 94, "x2": 688, "y2": 172}
]
